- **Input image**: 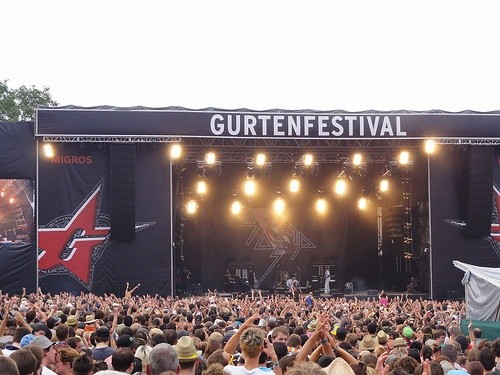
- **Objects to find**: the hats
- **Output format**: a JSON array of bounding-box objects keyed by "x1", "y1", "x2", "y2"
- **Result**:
[
  {"x1": 116, "y1": 334, "x2": 138, "y2": 349},
  {"x1": 375, "y1": 330, "x2": 389, "y2": 344},
  {"x1": 403, "y1": 326, "x2": 413, "y2": 340},
  {"x1": 359, "y1": 334, "x2": 378, "y2": 351},
  {"x1": 30, "y1": 335, "x2": 56, "y2": 350},
  {"x1": 20, "y1": 334, "x2": 36, "y2": 348},
  {"x1": 172, "y1": 336, "x2": 202, "y2": 360},
  {"x1": 64, "y1": 315, "x2": 80, "y2": 325},
  {"x1": 84, "y1": 315, "x2": 96, "y2": 323},
  {"x1": 392, "y1": 338, "x2": 407, "y2": 347},
  {"x1": 307, "y1": 320, "x2": 317, "y2": 329}
]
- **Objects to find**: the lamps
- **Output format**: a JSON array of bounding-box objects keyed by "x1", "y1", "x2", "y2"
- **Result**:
[
  {"x1": 293, "y1": 153, "x2": 319, "y2": 179},
  {"x1": 195, "y1": 152, "x2": 223, "y2": 178},
  {"x1": 272, "y1": 185, "x2": 285, "y2": 213},
  {"x1": 343, "y1": 152, "x2": 367, "y2": 183},
  {"x1": 389, "y1": 151, "x2": 415, "y2": 179},
  {"x1": 423, "y1": 246, "x2": 430, "y2": 254},
  {"x1": 246, "y1": 154, "x2": 273, "y2": 181}
]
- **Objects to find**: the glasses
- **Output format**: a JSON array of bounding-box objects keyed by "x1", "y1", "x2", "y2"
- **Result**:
[{"x1": 227, "y1": 354, "x2": 234, "y2": 365}]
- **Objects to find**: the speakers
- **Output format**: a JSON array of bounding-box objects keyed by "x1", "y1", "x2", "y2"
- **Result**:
[
  {"x1": 461, "y1": 146, "x2": 495, "y2": 238},
  {"x1": 332, "y1": 293, "x2": 344, "y2": 298},
  {"x1": 285, "y1": 291, "x2": 293, "y2": 297},
  {"x1": 232, "y1": 292, "x2": 241, "y2": 299},
  {"x1": 368, "y1": 290, "x2": 378, "y2": 296},
  {"x1": 108, "y1": 143, "x2": 136, "y2": 242}
]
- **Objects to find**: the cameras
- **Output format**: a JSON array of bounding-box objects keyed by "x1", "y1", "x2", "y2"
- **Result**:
[{"x1": 253, "y1": 319, "x2": 264, "y2": 326}]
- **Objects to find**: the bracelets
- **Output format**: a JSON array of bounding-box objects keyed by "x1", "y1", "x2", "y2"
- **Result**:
[
  {"x1": 333, "y1": 345, "x2": 338, "y2": 353},
  {"x1": 321, "y1": 339, "x2": 328, "y2": 345},
  {"x1": 273, "y1": 362, "x2": 279, "y2": 366},
  {"x1": 468, "y1": 327, "x2": 471, "y2": 331}
]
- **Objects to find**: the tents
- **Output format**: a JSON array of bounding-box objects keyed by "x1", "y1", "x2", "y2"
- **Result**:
[
  {"x1": 452, "y1": 261, "x2": 500, "y2": 341},
  {"x1": 0, "y1": 105, "x2": 500, "y2": 301}
]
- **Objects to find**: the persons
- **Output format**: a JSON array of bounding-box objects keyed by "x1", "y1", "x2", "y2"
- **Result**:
[
  {"x1": 0, "y1": 275, "x2": 500, "y2": 375},
  {"x1": 248, "y1": 267, "x2": 256, "y2": 291},
  {"x1": 324, "y1": 268, "x2": 330, "y2": 294}
]
